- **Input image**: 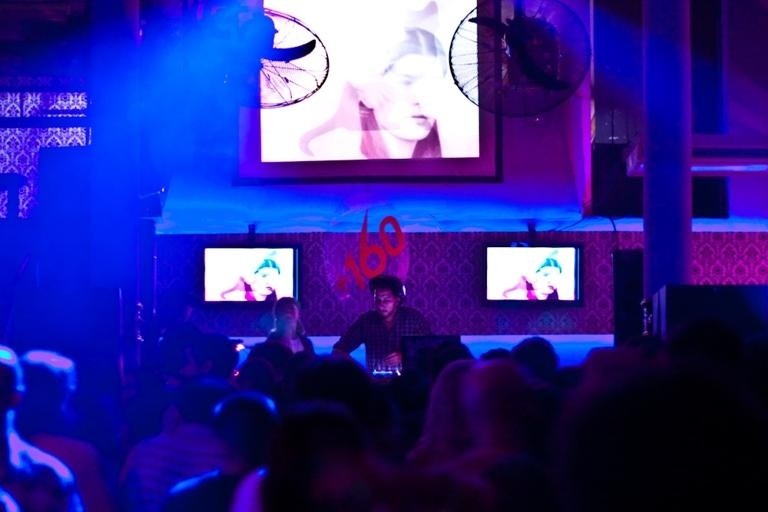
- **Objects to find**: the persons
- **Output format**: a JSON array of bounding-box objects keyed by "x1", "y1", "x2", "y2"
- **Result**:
[
  {"x1": 502, "y1": 257, "x2": 563, "y2": 300},
  {"x1": 331, "y1": 276, "x2": 434, "y2": 377},
  {"x1": 221, "y1": 258, "x2": 281, "y2": 302},
  {"x1": 266, "y1": 298, "x2": 315, "y2": 357},
  {"x1": 300, "y1": 25, "x2": 447, "y2": 161}
]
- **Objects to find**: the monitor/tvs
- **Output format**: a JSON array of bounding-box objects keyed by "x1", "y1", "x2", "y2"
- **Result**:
[
  {"x1": 200, "y1": 242, "x2": 299, "y2": 307},
  {"x1": 482, "y1": 241, "x2": 581, "y2": 306}
]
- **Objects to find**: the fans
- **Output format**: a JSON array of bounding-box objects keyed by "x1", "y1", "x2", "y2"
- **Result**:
[
  {"x1": 229, "y1": 8, "x2": 332, "y2": 111},
  {"x1": 449, "y1": 0, "x2": 593, "y2": 119}
]
